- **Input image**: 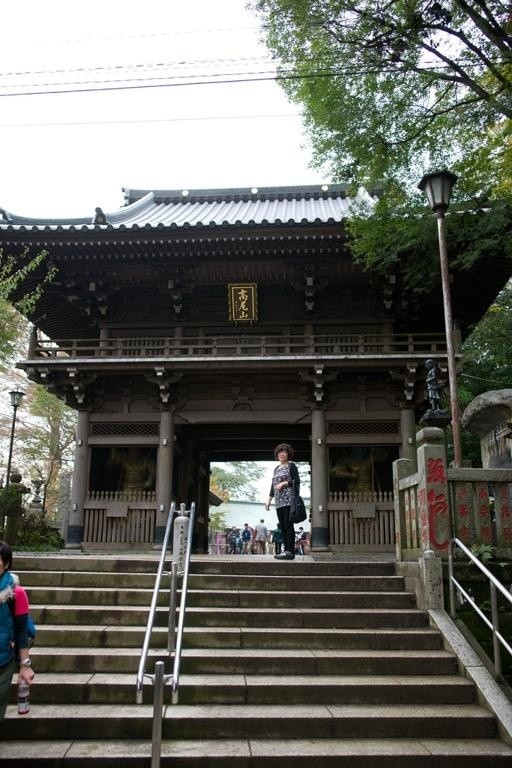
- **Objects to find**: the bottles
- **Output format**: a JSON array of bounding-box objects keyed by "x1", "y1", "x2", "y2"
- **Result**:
[{"x1": 16, "y1": 679, "x2": 30, "y2": 716}]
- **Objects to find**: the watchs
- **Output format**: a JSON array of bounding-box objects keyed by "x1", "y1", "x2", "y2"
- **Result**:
[{"x1": 19, "y1": 658, "x2": 32, "y2": 667}]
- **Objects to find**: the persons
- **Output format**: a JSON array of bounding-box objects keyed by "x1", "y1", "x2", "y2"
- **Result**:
[
  {"x1": 208, "y1": 519, "x2": 306, "y2": 556},
  {"x1": 265, "y1": 442, "x2": 299, "y2": 559},
  {"x1": 0, "y1": 540, "x2": 35, "y2": 731}
]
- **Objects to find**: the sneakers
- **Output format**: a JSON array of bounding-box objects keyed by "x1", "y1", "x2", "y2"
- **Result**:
[{"x1": 274, "y1": 551, "x2": 295, "y2": 559}]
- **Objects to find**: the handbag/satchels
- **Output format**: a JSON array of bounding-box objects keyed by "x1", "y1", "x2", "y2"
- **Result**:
[{"x1": 288, "y1": 479, "x2": 307, "y2": 524}]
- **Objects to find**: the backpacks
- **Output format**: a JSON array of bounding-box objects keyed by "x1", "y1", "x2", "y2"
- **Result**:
[{"x1": 6, "y1": 597, "x2": 35, "y2": 663}]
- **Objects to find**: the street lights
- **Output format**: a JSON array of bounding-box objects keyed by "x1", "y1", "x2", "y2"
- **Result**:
[
  {"x1": 0, "y1": 387, "x2": 25, "y2": 543},
  {"x1": 414, "y1": 166, "x2": 463, "y2": 466}
]
